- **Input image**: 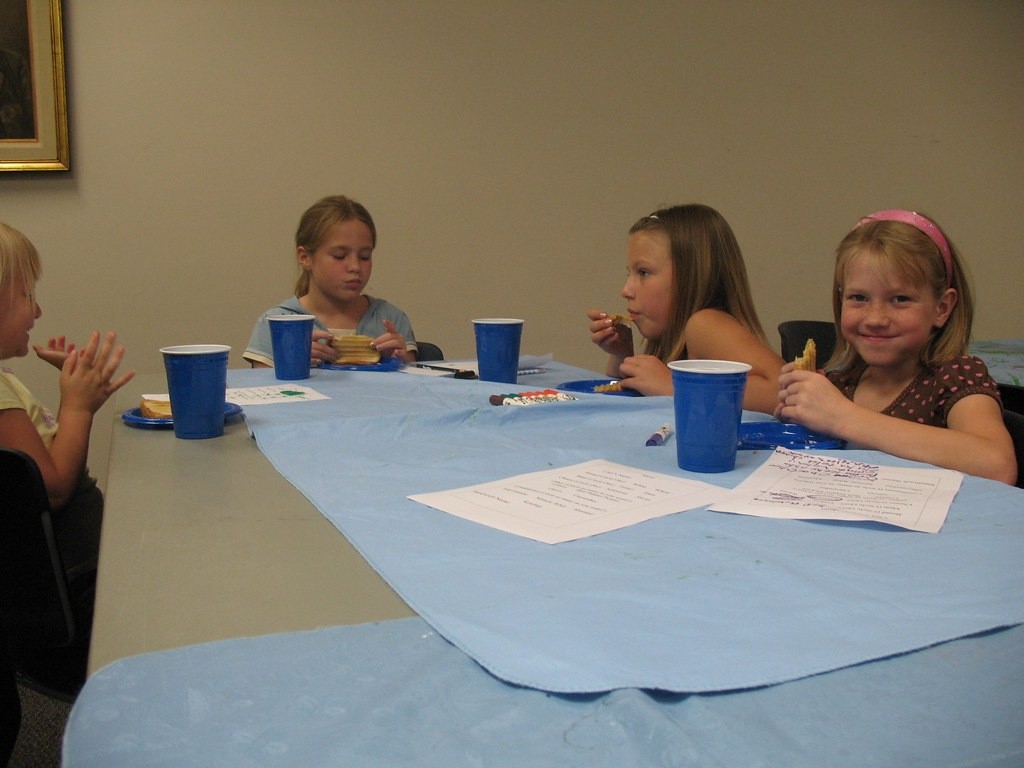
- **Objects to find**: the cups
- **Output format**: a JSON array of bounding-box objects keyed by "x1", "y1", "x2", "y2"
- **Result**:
[
  {"x1": 470, "y1": 318, "x2": 526, "y2": 384},
  {"x1": 666, "y1": 359, "x2": 752, "y2": 474},
  {"x1": 266, "y1": 315, "x2": 316, "y2": 380},
  {"x1": 158, "y1": 345, "x2": 232, "y2": 439}
]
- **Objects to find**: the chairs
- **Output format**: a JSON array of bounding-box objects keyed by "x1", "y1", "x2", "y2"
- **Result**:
[
  {"x1": 0, "y1": 447, "x2": 90, "y2": 703},
  {"x1": 777, "y1": 321, "x2": 836, "y2": 370},
  {"x1": 996, "y1": 383, "x2": 1024, "y2": 489}
]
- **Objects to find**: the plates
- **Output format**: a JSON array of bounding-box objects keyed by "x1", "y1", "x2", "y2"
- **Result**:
[
  {"x1": 737, "y1": 421, "x2": 848, "y2": 450},
  {"x1": 555, "y1": 380, "x2": 640, "y2": 397},
  {"x1": 317, "y1": 357, "x2": 402, "y2": 372},
  {"x1": 121, "y1": 401, "x2": 243, "y2": 425}
]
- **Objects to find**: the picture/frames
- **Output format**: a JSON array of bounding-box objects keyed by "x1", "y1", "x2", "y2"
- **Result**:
[{"x1": 0, "y1": 0, "x2": 70, "y2": 172}]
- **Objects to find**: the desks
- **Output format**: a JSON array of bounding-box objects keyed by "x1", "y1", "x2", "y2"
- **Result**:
[{"x1": 77, "y1": 357, "x2": 1024, "y2": 767}]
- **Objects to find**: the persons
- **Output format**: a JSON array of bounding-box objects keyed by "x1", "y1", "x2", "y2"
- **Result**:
[
  {"x1": 586, "y1": 202, "x2": 791, "y2": 414},
  {"x1": 772, "y1": 208, "x2": 1020, "y2": 483},
  {"x1": 243, "y1": 194, "x2": 418, "y2": 370},
  {"x1": 0, "y1": 223, "x2": 136, "y2": 516}
]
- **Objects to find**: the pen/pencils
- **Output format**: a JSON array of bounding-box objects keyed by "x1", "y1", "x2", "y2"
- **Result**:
[{"x1": 410, "y1": 363, "x2": 460, "y2": 373}]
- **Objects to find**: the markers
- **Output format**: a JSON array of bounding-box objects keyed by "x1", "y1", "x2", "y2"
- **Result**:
[
  {"x1": 646, "y1": 424, "x2": 673, "y2": 447},
  {"x1": 490, "y1": 389, "x2": 576, "y2": 406}
]
